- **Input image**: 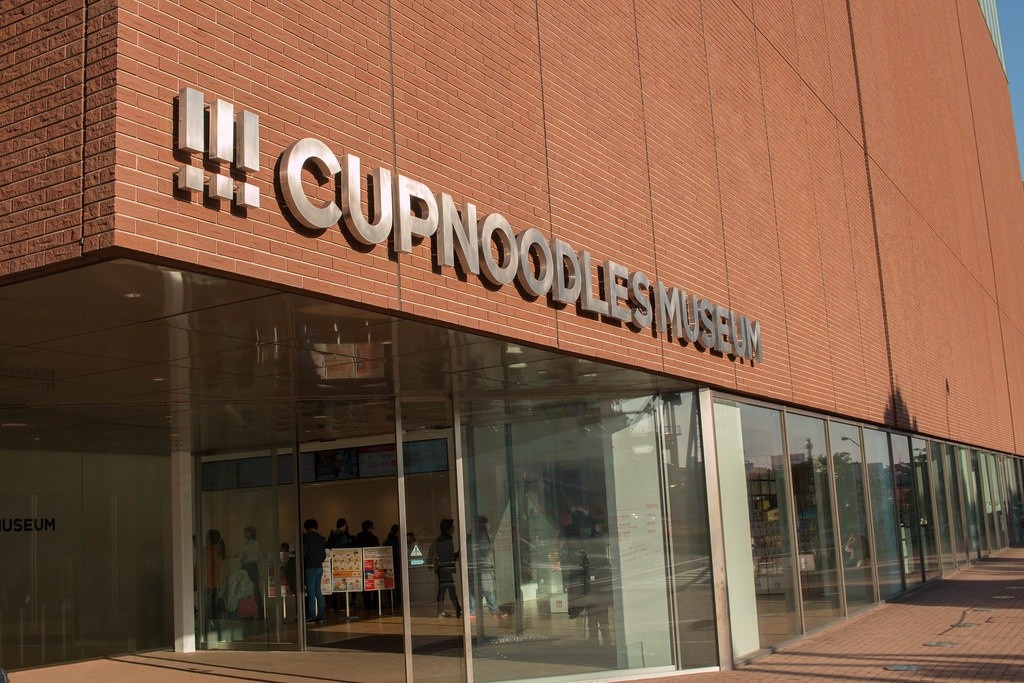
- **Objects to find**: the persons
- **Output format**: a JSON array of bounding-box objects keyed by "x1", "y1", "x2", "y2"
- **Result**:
[
  {"x1": 280, "y1": 543, "x2": 289, "y2": 552},
  {"x1": 326, "y1": 518, "x2": 355, "y2": 612},
  {"x1": 354, "y1": 520, "x2": 380, "y2": 611},
  {"x1": 194, "y1": 529, "x2": 226, "y2": 643},
  {"x1": 567, "y1": 517, "x2": 613, "y2": 650},
  {"x1": 468, "y1": 516, "x2": 508, "y2": 619},
  {"x1": 382, "y1": 525, "x2": 403, "y2": 609},
  {"x1": 238, "y1": 525, "x2": 264, "y2": 624},
  {"x1": 303, "y1": 519, "x2": 326, "y2": 624},
  {"x1": 407, "y1": 532, "x2": 419, "y2": 561},
  {"x1": 436, "y1": 518, "x2": 462, "y2": 618}
]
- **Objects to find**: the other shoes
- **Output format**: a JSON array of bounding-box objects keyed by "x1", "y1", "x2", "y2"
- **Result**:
[
  {"x1": 457, "y1": 610, "x2": 463, "y2": 617},
  {"x1": 439, "y1": 612, "x2": 451, "y2": 618},
  {"x1": 304, "y1": 617, "x2": 324, "y2": 625}
]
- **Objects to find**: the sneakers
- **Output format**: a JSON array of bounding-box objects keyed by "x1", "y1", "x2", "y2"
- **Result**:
[
  {"x1": 468, "y1": 613, "x2": 477, "y2": 619},
  {"x1": 493, "y1": 610, "x2": 508, "y2": 619}
]
